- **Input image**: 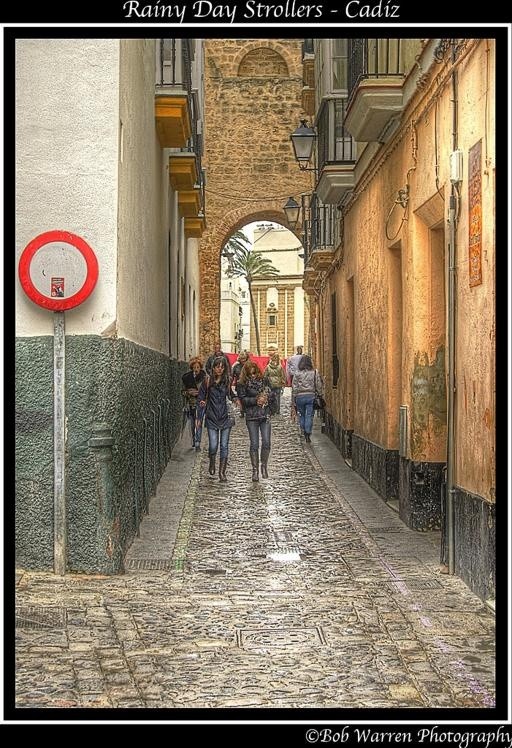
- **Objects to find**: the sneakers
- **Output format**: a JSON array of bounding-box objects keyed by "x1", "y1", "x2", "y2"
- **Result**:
[{"x1": 191, "y1": 443, "x2": 202, "y2": 452}]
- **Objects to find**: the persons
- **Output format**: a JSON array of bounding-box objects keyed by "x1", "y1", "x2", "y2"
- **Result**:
[
  {"x1": 205, "y1": 343, "x2": 229, "y2": 382},
  {"x1": 291, "y1": 355, "x2": 324, "y2": 443},
  {"x1": 287, "y1": 346, "x2": 307, "y2": 383},
  {"x1": 234, "y1": 360, "x2": 277, "y2": 482},
  {"x1": 193, "y1": 357, "x2": 241, "y2": 481},
  {"x1": 229, "y1": 350, "x2": 254, "y2": 419},
  {"x1": 181, "y1": 358, "x2": 208, "y2": 452},
  {"x1": 263, "y1": 354, "x2": 286, "y2": 414}
]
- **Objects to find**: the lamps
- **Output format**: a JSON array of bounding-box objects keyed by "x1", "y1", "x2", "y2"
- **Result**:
[
  {"x1": 282, "y1": 196, "x2": 319, "y2": 236},
  {"x1": 289, "y1": 117, "x2": 322, "y2": 172}
]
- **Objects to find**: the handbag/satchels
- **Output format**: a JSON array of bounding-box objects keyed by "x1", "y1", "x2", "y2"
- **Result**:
[{"x1": 313, "y1": 398, "x2": 327, "y2": 410}]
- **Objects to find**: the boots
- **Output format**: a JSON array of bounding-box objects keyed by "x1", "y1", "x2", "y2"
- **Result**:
[
  {"x1": 208, "y1": 453, "x2": 216, "y2": 475},
  {"x1": 218, "y1": 462, "x2": 227, "y2": 482},
  {"x1": 249, "y1": 451, "x2": 260, "y2": 482},
  {"x1": 305, "y1": 432, "x2": 312, "y2": 443},
  {"x1": 261, "y1": 447, "x2": 271, "y2": 479}
]
